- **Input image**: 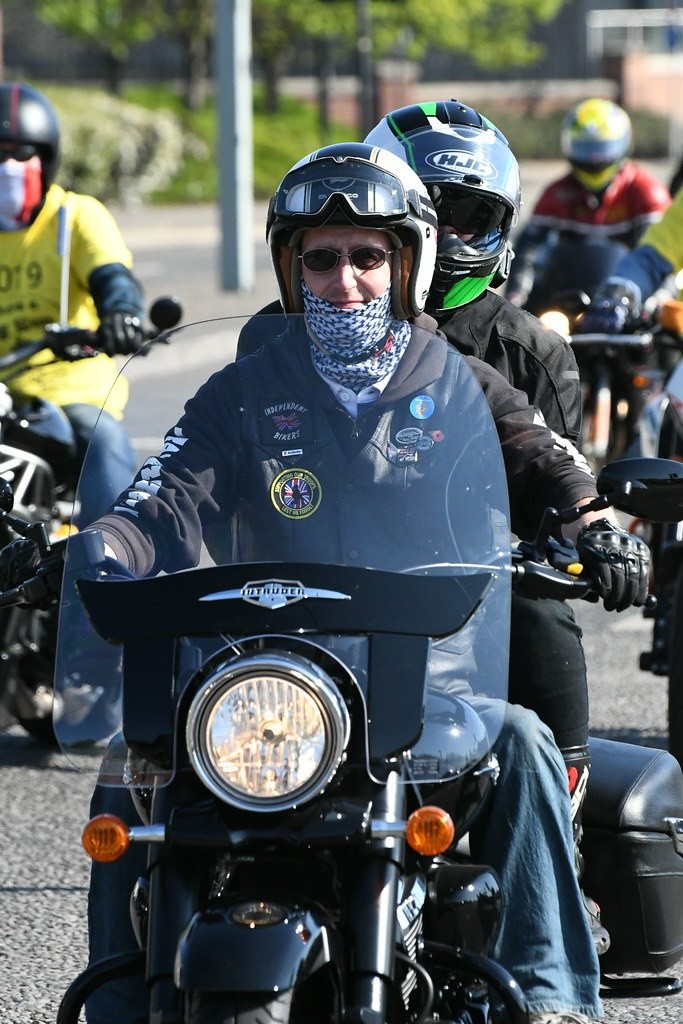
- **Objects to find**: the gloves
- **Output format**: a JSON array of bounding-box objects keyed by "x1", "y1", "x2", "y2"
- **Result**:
[
  {"x1": 574, "y1": 516, "x2": 654, "y2": 611},
  {"x1": 95, "y1": 309, "x2": 152, "y2": 360}
]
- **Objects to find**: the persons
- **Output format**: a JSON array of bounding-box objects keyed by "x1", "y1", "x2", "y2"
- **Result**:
[
  {"x1": 0, "y1": 77, "x2": 161, "y2": 745},
  {"x1": 59, "y1": 137, "x2": 612, "y2": 1024},
  {"x1": 514, "y1": 92, "x2": 666, "y2": 284},
  {"x1": 343, "y1": 102, "x2": 642, "y2": 828}
]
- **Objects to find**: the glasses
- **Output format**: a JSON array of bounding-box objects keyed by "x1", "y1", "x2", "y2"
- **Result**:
[
  {"x1": 295, "y1": 245, "x2": 395, "y2": 272},
  {"x1": 1, "y1": 139, "x2": 50, "y2": 167},
  {"x1": 433, "y1": 193, "x2": 508, "y2": 236}
]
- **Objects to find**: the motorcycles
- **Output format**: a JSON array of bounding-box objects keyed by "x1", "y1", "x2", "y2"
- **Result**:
[
  {"x1": 500, "y1": 237, "x2": 676, "y2": 538},
  {"x1": 2, "y1": 307, "x2": 683, "y2": 1024},
  {"x1": 541, "y1": 302, "x2": 682, "y2": 762},
  {"x1": 0, "y1": 288, "x2": 187, "y2": 754}
]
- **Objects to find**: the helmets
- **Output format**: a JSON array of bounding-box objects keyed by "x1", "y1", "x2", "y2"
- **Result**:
[
  {"x1": 0, "y1": 83, "x2": 61, "y2": 226},
  {"x1": 362, "y1": 101, "x2": 523, "y2": 316},
  {"x1": 559, "y1": 98, "x2": 634, "y2": 193},
  {"x1": 265, "y1": 141, "x2": 438, "y2": 322}
]
